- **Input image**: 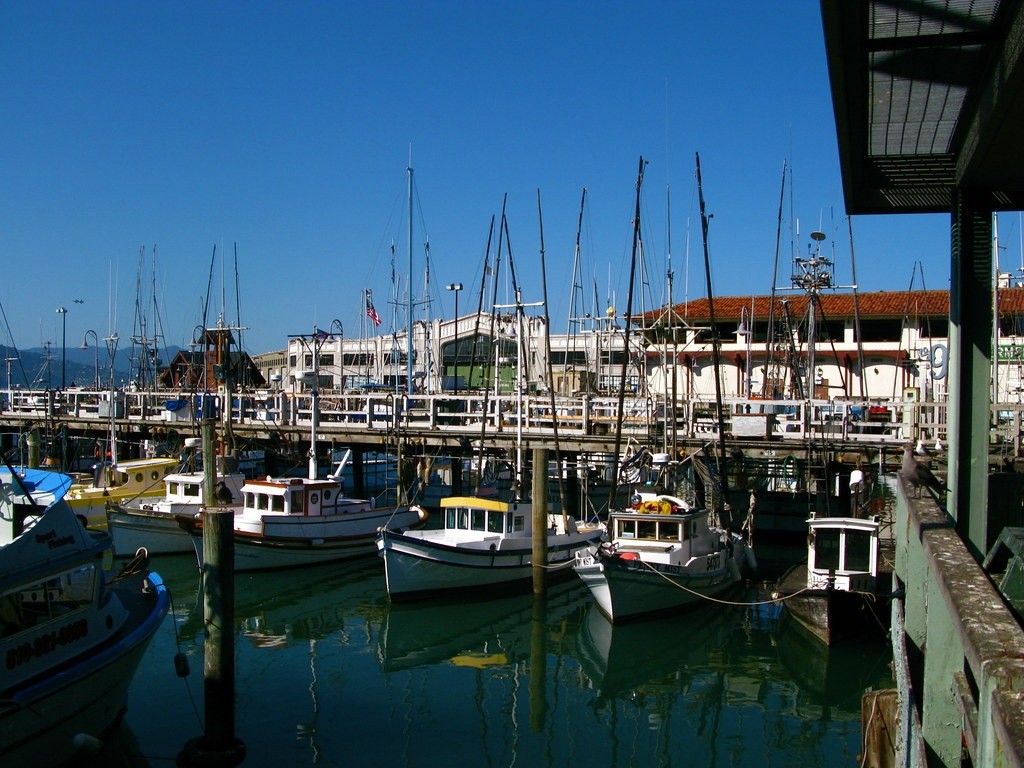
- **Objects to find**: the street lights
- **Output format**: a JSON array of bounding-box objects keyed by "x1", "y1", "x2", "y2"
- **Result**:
[
  {"x1": 503, "y1": 305, "x2": 530, "y2": 428},
  {"x1": 55, "y1": 306, "x2": 67, "y2": 394},
  {"x1": 444, "y1": 282, "x2": 464, "y2": 395},
  {"x1": 188, "y1": 324, "x2": 209, "y2": 394},
  {"x1": 78, "y1": 329, "x2": 101, "y2": 407},
  {"x1": 733, "y1": 302, "x2": 753, "y2": 403},
  {"x1": 324, "y1": 318, "x2": 344, "y2": 412}
]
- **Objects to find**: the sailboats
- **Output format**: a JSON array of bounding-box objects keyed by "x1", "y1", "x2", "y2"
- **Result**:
[{"x1": 1, "y1": 150, "x2": 895, "y2": 760}]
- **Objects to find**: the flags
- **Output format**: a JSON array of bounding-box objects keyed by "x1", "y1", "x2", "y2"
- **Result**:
[{"x1": 366, "y1": 298, "x2": 381, "y2": 327}]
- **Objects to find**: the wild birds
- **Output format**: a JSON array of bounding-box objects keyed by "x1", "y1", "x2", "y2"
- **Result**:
[
  {"x1": 214, "y1": 481, "x2": 235, "y2": 511},
  {"x1": 896, "y1": 438, "x2": 945, "y2": 499}
]
